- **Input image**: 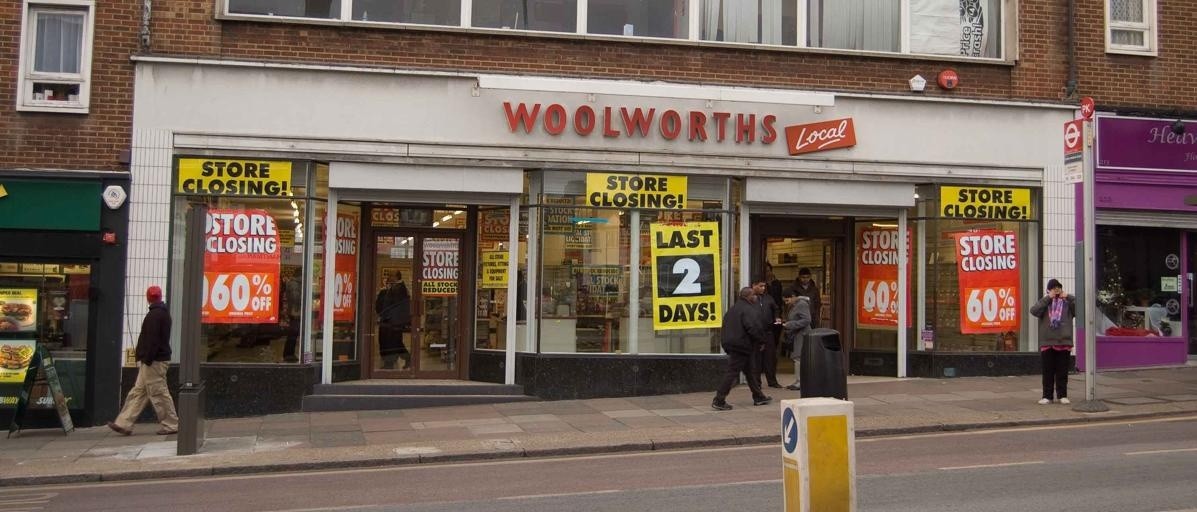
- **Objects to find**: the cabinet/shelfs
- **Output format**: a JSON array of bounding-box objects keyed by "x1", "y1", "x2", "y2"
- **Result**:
[
  {"x1": 423, "y1": 265, "x2": 670, "y2": 365},
  {"x1": 1117, "y1": 306, "x2": 1168, "y2": 333}
]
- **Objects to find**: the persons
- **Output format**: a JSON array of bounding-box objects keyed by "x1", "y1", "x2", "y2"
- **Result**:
[
  {"x1": 751, "y1": 278, "x2": 782, "y2": 389},
  {"x1": 711, "y1": 286, "x2": 774, "y2": 410},
  {"x1": 766, "y1": 262, "x2": 783, "y2": 362},
  {"x1": 517, "y1": 271, "x2": 527, "y2": 322},
  {"x1": 784, "y1": 267, "x2": 823, "y2": 329},
  {"x1": 375, "y1": 268, "x2": 411, "y2": 369},
  {"x1": 1029, "y1": 278, "x2": 1075, "y2": 405},
  {"x1": 283, "y1": 266, "x2": 302, "y2": 361},
  {"x1": 108, "y1": 285, "x2": 180, "y2": 436},
  {"x1": 780, "y1": 288, "x2": 813, "y2": 390}
]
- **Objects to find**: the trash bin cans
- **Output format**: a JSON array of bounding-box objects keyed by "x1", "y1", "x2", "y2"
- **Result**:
[{"x1": 799, "y1": 328, "x2": 848, "y2": 401}]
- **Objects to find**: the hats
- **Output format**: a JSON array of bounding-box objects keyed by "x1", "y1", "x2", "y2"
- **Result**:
[
  {"x1": 147, "y1": 286, "x2": 162, "y2": 304},
  {"x1": 1047, "y1": 279, "x2": 1062, "y2": 293},
  {"x1": 799, "y1": 267, "x2": 810, "y2": 275}
]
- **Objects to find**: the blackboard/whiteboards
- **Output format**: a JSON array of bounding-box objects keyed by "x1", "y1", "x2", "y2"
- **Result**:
[{"x1": 38, "y1": 346, "x2": 74, "y2": 434}]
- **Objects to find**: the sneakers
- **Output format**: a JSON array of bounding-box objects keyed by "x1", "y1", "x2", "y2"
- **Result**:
[
  {"x1": 787, "y1": 385, "x2": 800, "y2": 390},
  {"x1": 403, "y1": 359, "x2": 411, "y2": 369},
  {"x1": 108, "y1": 421, "x2": 132, "y2": 435},
  {"x1": 1058, "y1": 397, "x2": 1069, "y2": 404},
  {"x1": 754, "y1": 396, "x2": 772, "y2": 405},
  {"x1": 769, "y1": 383, "x2": 781, "y2": 388},
  {"x1": 712, "y1": 397, "x2": 732, "y2": 410},
  {"x1": 1038, "y1": 398, "x2": 1054, "y2": 404},
  {"x1": 157, "y1": 429, "x2": 177, "y2": 434}
]
